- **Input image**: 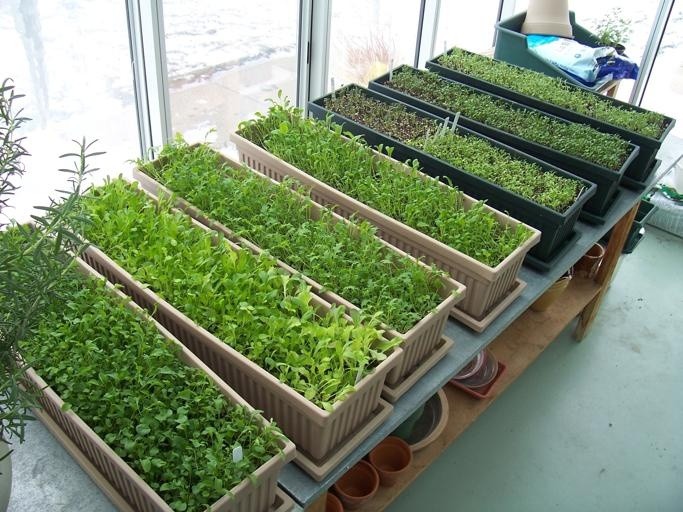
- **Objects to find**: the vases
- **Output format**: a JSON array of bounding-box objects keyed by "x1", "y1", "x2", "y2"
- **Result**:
[
  {"x1": 324, "y1": 493, "x2": 343, "y2": 512},
  {"x1": 451, "y1": 358, "x2": 506, "y2": 397},
  {"x1": 335, "y1": 457, "x2": 380, "y2": 508},
  {"x1": 394, "y1": 388, "x2": 449, "y2": 453},
  {"x1": 369, "y1": 437, "x2": 412, "y2": 487},
  {"x1": 604, "y1": 201, "x2": 659, "y2": 253},
  {"x1": 576, "y1": 244, "x2": 604, "y2": 279},
  {"x1": 520, "y1": 0, "x2": 572, "y2": 38},
  {"x1": 531, "y1": 275, "x2": 571, "y2": 313}
]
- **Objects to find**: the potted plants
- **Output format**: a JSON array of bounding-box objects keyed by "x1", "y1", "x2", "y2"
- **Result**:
[
  {"x1": 308, "y1": 82, "x2": 596, "y2": 261},
  {"x1": 368, "y1": 63, "x2": 638, "y2": 213},
  {"x1": 44, "y1": 181, "x2": 403, "y2": 463},
  {"x1": 426, "y1": 48, "x2": 677, "y2": 182},
  {"x1": 132, "y1": 142, "x2": 466, "y2": 385},
  {"x1": 0, "y1": 222, "x2": 299, "y2": 512},
  {"x1": 228, "y1": 89, "x2": 541, "y2": 319}
]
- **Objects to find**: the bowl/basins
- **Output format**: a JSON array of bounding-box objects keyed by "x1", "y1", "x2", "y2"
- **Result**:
[{"x1": 410, "y1": 388, "x2": 450, "y2": 452}]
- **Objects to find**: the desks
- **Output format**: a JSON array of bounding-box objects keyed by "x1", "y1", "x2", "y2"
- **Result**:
[{"x1": 0, "y1": 150, "x2": 683, "y2": 511}]
